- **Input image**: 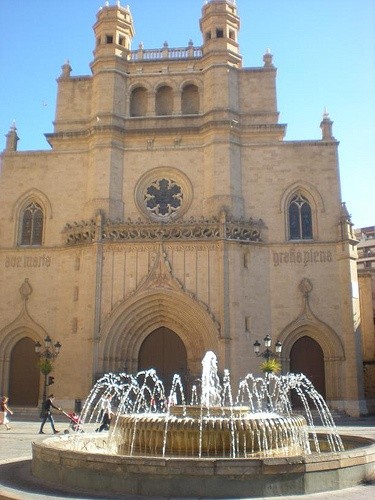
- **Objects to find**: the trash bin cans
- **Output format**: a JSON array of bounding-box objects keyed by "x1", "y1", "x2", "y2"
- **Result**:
[{"x1": 75, "y1": 399, "x2": 81, "y2": 412}]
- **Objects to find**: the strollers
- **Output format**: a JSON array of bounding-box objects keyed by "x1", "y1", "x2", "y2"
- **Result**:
[{"x1": 60, "y1": 409, "x2": 85, "y2": 433}]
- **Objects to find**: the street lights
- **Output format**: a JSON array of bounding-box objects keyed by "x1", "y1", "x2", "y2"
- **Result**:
[
  {"x1": 253, "y1": 335, "x2": 282, "y2": 414},
  {"x1": 34, "y1": 336, "x2": 62, "y2": 418}
]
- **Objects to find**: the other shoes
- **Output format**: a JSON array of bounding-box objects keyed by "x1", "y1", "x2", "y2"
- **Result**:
[
  {"x1": 54, "y1": 431, "x2": 59, "y2": 434},
  {"x1": 7, "y1": 428, "x2": 11, "y2": 430},
  {"x1": 39, "y1": 431, "x2": 45, "y2": 434}
]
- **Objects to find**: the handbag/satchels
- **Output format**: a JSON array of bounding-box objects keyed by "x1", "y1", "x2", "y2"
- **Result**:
[{"x1": 40, "y1": 411, "x2": 50, "y2": 418}]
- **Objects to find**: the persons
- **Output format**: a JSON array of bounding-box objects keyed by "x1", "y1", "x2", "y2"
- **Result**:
[
  {"x1": 72, "y1": 413, "x2": 81, "y2": 423},
  {"x1": 0, "y1": 395, "x2": 14, "y2": 431},
  {"x1": 38, "y1": 394, "x2": 60, "y2": 434},
  {"x1": 93, "y1": 392, "x2": 179, "y2": 436}
]
